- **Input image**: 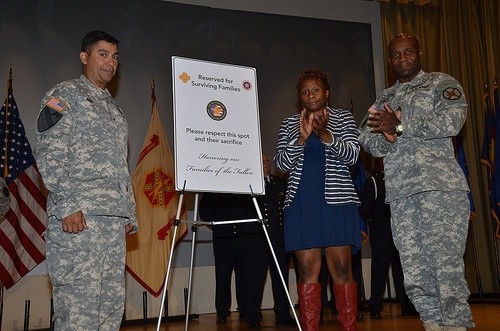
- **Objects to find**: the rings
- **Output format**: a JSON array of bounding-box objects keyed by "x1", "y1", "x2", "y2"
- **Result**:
[{"x1": 382, "y1": 122, "x2": 384, "y2": 126}]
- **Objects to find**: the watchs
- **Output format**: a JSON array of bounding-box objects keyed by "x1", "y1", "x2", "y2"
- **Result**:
[{"x1": 395, "y1": 120, "x2": 402, "y2": 137}]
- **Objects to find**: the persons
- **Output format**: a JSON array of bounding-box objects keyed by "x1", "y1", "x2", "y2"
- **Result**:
[
  {"x1": 199, "y1": 153, "x2": 418, "y2": 329},
  {"x1": 360, "y1": 32, "x2": 478, "y2": 331},
  {"x1": 34, "y1": 31, "x2": 138, "y2": 330},
  {"x1": 275, "y1": 68, "x2": 363, "y2": 331}
]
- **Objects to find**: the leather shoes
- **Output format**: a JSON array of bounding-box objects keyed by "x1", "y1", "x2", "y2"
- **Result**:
[
  {"x1": 276, "y1": 317, "x2": 298, "y2": 327},
  {"x1": 250, "y1": 320, "x2": 262, "y2": 330},
  {"x1": 239, "y1": 312, "x2": 250, "y2": 322},
  {"x1": 216, "y1": 316, "x2": 227, "y2": 324},
  {"x1": 370, "y1": 310, "x2": 382, "y2": 318},
  {"x1": 401, "y1": 308, "x2": 419, "y2": 315}
]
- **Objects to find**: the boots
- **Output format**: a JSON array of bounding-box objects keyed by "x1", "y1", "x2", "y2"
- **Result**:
[
  {"x1": 333, "y1": 283, "x2": 359, "y2": 331},
  {"x1": 297, "y1": 281, "x2": 322, "y2": 331}
]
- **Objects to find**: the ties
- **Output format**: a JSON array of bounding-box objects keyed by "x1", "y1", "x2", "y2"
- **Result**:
[{"x1": 264, "y1": 177, "x2": 272, "y2": 193}]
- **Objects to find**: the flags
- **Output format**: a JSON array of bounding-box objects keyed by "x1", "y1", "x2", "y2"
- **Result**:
[
  {"x1": 0, "y1": 88, "x2": 51, "y2": 291},
  {"x1": 124, "y1": 90, "x2": 188, "y2": 298},
  {"x1": 455, "y1": 82, "x2": 500, "y2": 239}
]
